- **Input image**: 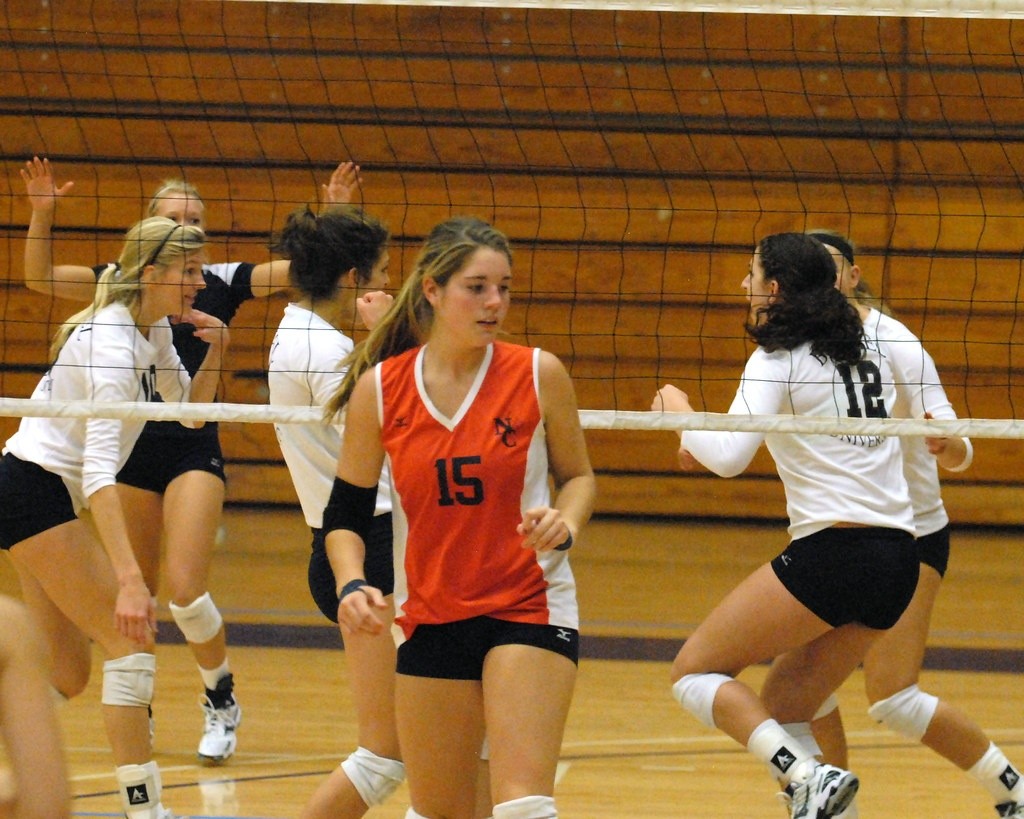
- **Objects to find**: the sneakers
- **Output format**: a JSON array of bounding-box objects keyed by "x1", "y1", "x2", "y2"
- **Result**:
[
  {"x1": 197, "y1": 693, "x2": 242, "y2": 762},
  {"x1": 774, "y1": 762, "x2": 860, "y2": 819},
  {"x1": 993, "y1": 798, "x2": 1024, "y2": 819}
]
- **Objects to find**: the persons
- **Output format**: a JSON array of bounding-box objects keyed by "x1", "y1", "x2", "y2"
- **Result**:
[
  {"x1": 320, "y1": 215, "x2": 598, "y2": 819},
  {"x1": 0, "y1": 217, "x2": 230, "y2": 819},
  {"x1": 810, "y1": 233, "x2": 1024, "y2": 819},
  {"x1": 649, "y1": 235, "x2": 918, "y2": 819},
  {"x1": 266, "y1": 202, "x2": 494, "y2": 819},
  {"x1": 20, "y1": 156, "x2": 367, "y2": 761}
]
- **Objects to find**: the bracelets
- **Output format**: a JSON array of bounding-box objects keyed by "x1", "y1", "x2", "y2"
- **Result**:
[
  {"x1": 339, "y1": 579, "x2": 369, "y2": 603},
  {"x1": 553, "y1": 525, "x2": 572, "y2": 551}
]
